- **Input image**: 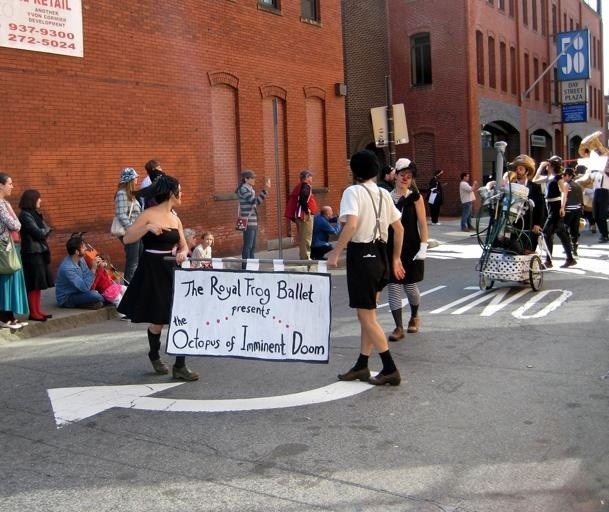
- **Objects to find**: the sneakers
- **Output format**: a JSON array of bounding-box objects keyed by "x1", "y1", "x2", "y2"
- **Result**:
[
  {"x1": 0, "y1": 320, "x2": 22, "y2": 329},
  {"x1": 15, "y1": 320, "x2": 28, "y2": 326}
]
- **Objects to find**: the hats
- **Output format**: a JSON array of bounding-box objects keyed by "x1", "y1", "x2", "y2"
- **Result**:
[
  {"x1": 546, "y1": 156, "x2": 563, "y2": 168},
  {"x1": 395, "y1": 158, "x2": 417, "y2": 174},
  {"x1": 560, "y1": 168, "x2": 575, "y2": 174},
  {"x1": 507, "y1": 154, "x2": 535, "y2": 179},
  {"x1": 118, "y1": 168, "x2": 140, "y2": 182},
  {"x1": 300, "y1": 170, "x2": 316, "y2": 178},
  {"x1": 241, "y1": 170, "x2": 257, "y2": 179}
]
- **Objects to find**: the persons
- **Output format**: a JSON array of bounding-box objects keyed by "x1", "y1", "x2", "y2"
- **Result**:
[
  {"x1": 112, "y1": 167, "x2": 144, "y2": 287},
  {"x1": 311, "y1": 205, "x2": 343, "y2": 260},
  {"x1": 459, "y1": 171, "x2": 480, "y2": 232},
  {"x1": 531, "y1": 155, "x2": 577, "y2": 269},
  {"x1": 386, "y1": 156, "x2": 429, "y2": 341},
  {"x1": 0, "y1": 173, "x2": 31, "y2": 331},
  {"x1": 324, "y1": 147, "x2": 408, "y2": 387},
  {"x1": 561, "y1": 168, "x2": 584, "y2": 257},
  {"x1": 190, "y1": 230, "x2": 215, "y2": 269},
  {"x1": 234, "y1": 169, "x2": 272, "y2": 271},
  {"x1": 497, "y1": 155, "x2": 547, "y2": 283},
  {"x1": 589, "y1": 169, "x2": 609, "y2": 244},
  {"x1": 426, "y1": 168, "x2": 446, "y2": 226},
  {"x1": 575, "y1": 165, "x2": 596, "y2": 233},
  {"x1": 54, "y1": 236, "x2": 107, "y2": 310},
  {"x1": 379, "y1": 163, "x2": 398, "y2": 190},
  {"x1": 139, "y1": 159, "x2": 163, "y2": 191},
  {"x1": 284, "y1": 171, "x2": 318, "y2": 267},
  {"x1": 17, "y1": 188, "x2": 55, "y2": 322},
  {"x1": 183, "y1": 227, "x2": 197, "y2": 256},
  {"x1": 116, "y1": 168, "x2": 200, "y2": 381},
  {"x1": 91, "y1": 252, "x2": 132, "y2": 323}
]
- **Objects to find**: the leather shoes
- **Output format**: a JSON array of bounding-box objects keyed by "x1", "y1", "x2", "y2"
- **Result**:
[
  {"x1": 337, "y1": 367, "x2": 370, "y2": 381},
  {"x1": 407, "y1": 317, "x2": 422, "y2": 332},
  {"x1": 468, "y1": 225, "x2": 477, "y2": 231},
  {"x1": 542, "y1": 261, "x2": 552, "y2": 269},
  {"x1": 388, "y1": 328, "x2": 405, "y2": 342},
  {"x1": 44, "y1": 315, "x2": 53, "y2": 318},
  {"x1": 561, "y1": 258, "x2": 576, "y2": 268},
  {"x1": 172, "y1": 364, "x2": 200, "y2": 381},
  {"x1": 28, "y1": 314, "x2": 46, "y2": 321},
  {"x1": 370, "y1": 370, "x2": 401, "y2": 386},
  {"x1": 149, "y1": 352, "x2": 169, "y2": 375},
  {"x1": 461, "y1": 227, "x2": 469, "y2": 231}
]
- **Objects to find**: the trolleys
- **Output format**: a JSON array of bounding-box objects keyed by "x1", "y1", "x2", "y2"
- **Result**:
[{"x1": 475, "y1": 227, "x2": 554, "y2": 292}]
